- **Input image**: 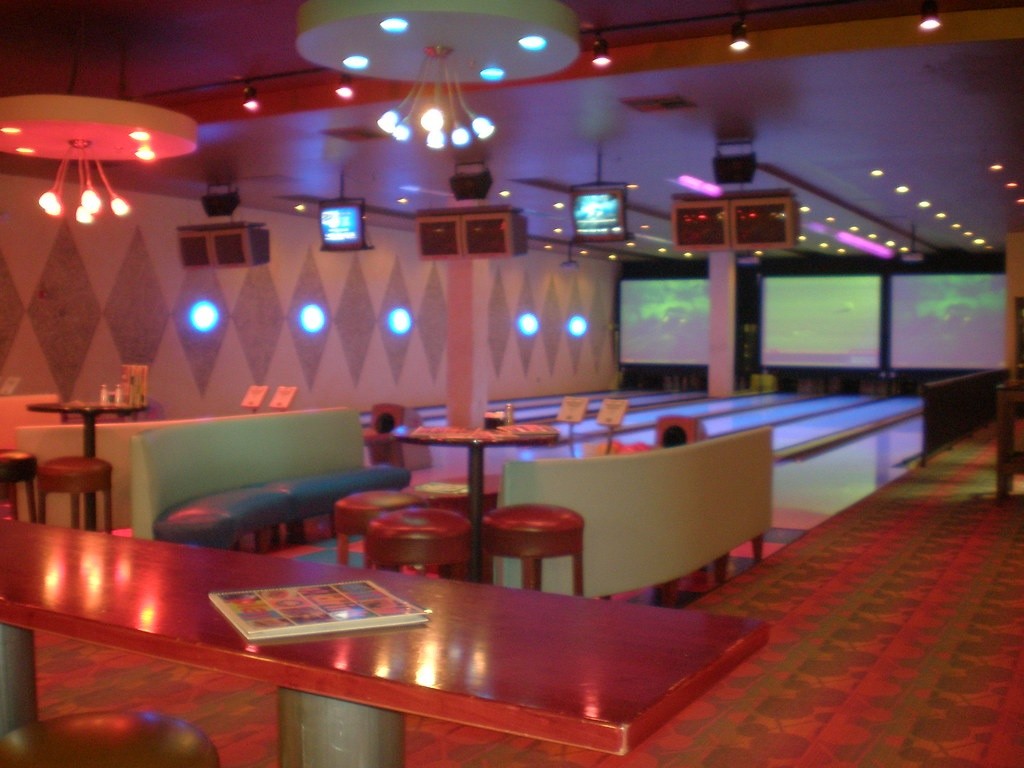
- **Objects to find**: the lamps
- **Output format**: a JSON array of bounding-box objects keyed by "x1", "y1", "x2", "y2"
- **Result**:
[
  {"x1": 0, "y1": 1, "x2": 200, "y2": 223},
  {"x1": 293, "y1": 1, "x2": 581, "y2": 149}
]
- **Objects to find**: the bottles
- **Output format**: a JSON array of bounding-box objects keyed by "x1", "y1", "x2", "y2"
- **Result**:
[
  {"x1": 100, "y1": 384, "x2": 108, "y2": 404},
  {"x1": 505, "y1": 404, "x2": 512, "y2": 425},
  {"x1": 129, "y1": 376, "x2": 142, "y2": 404},
  {"x1": 115, "y1": 384, "x2": 122, "y2": 404}
]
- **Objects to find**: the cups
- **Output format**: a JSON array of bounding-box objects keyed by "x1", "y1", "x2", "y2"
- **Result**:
[{"x1": 485, "y1": 418, "x2": 499, "y2": 429}]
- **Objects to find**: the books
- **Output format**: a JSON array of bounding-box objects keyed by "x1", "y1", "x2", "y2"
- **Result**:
[{"x1": 209, "y1": 580, "x2": 430, "y2": 641}]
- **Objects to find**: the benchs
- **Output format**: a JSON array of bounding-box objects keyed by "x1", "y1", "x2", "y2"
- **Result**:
[
  {"x1": 496, "y1": 425, "x2": 774, "y2": 608},
  {"x1": 13, "y1": 420, "x2": 180, "y2": 526},
  {"x1": 132, "y1": 408, "x2": 411, "y2": 555}
]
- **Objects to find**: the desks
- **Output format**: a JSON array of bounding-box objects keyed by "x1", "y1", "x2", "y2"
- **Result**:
[
  {"x1": 0, "y1": 526, "x2": 771, "y2": 767},
  {"x1": 393, "y1": 420, "x2": 560, "y2": 584},
  {"x1": 30, "y1": 399, "x2": 149, "y2": 534}
]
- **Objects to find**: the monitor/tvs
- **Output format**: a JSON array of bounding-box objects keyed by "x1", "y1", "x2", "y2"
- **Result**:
[
  {"x1": 570, "y1": 188, "x2": 635, "y2": 243},
  {"x1": 177, "y1": 230, "x2": 213, "y2": 270},
  {"x1": 210, "y1": 226, "x2": 270, "y2": 268},
  {"x1": 415, "y1": 216, "x2": 464, "y2": 261},
  {"x1": 319, "y1": 204, "x2": 365, "y2": 250},
  {"x1": 461, "y1": 212, "x2": 527, "y2": 258},
  {"x1": 730, "y1": 197, "x2": 801, "y2": 250},
  {"x1": 672, "y1": 200, "x2": 731, "y2": 251}
]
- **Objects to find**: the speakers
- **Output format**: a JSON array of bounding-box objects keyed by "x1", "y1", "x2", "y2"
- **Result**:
[
  {"x1": 201, "y1": 193, "x2": 240, "y2": 218},
  {"x1": 713, "y1": 151, "x2": 756, "y2": 184},
  {"x1": 448, "y1": 170, "x2": 492, "y2": 201}
]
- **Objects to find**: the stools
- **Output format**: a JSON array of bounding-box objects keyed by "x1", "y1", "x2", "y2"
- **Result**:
[
  {"x1": 0, "y1": 450, "x2": 36, "y2": 516},
  {"x1": 414, "y1": 482, "x2": 497, "y2": 520},
  {"x1": 481, "y1": 504, "x2": 585, "y2": 596},
  {"x1": 36, "y1": 455, "x2": 111, "y2": 537},
  {"x1": 367, "y1": 509, "x2": 473, "y2": 580},
  {"x1": 333, "y1": 492, "x2": 424, "y2": 568}
]
